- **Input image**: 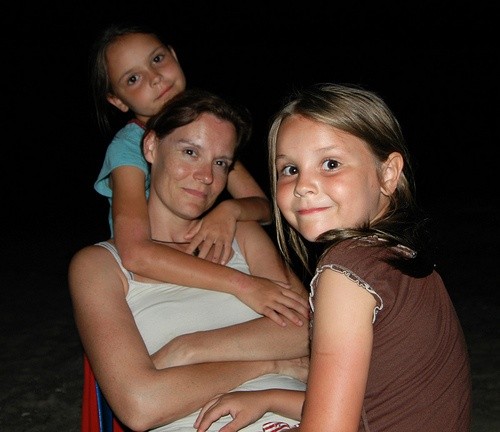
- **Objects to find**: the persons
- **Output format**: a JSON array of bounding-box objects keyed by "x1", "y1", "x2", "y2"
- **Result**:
[
  {"x1": 92, "y1": 22, "x2": 311, "y2": 328},
  {"x1": 192, "y1": 82, "x2": 473, "y2": 432},
  {"x1": 66, "y1": 87, "x2": 316, "y2": 432}
]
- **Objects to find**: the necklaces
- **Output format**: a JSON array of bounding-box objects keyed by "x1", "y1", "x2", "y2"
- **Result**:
[{"x1": 152, "y1": 239, "x2": 200, "y2": 256}]
